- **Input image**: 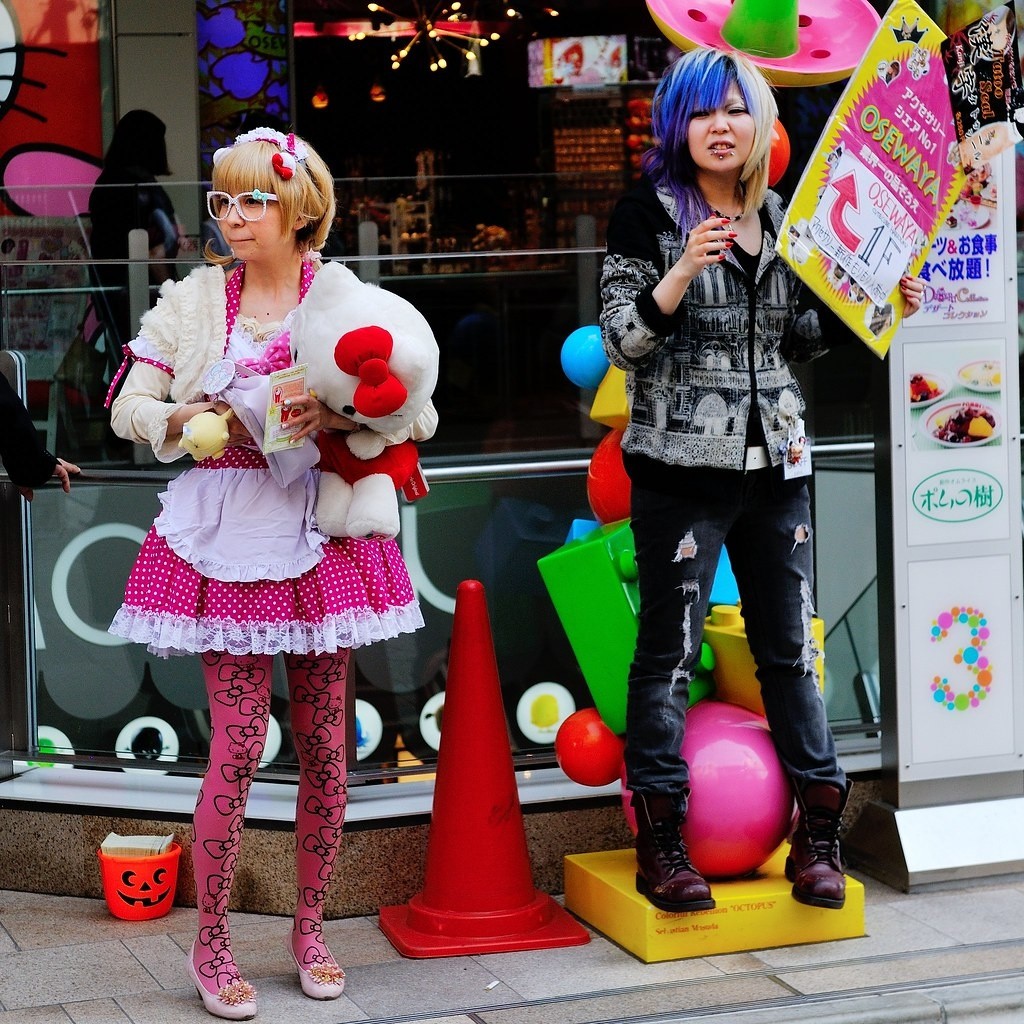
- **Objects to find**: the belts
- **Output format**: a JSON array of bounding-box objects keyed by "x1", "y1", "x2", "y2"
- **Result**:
[{"x1": 745, "y1": 446, "x2": 769, "y2": 471}]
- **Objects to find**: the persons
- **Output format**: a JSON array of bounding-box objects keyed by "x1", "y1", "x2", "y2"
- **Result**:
[
  {"x1": 1, "y1": 371, "x2": 80, "y2": 501},
  {"x1": 100, "y1": 132, "x2": 442, "y2": 1014},
  {"x1": 84, "y1": 108, "x2": 180, "y2": 465},
  {"x1": 598, "y1": 46, "x2": 926, "y2": 910}
]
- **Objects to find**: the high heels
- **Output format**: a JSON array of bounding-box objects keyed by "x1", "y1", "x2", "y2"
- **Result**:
[
  {"x1": 186, "y1": 939, "x2": 258, "y2": 1021},
  {"x1": 286, "y1": 926, "x2": 346, "y2": 1001}
]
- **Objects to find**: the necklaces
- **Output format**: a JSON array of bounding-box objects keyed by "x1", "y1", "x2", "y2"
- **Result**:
[{"x1": 705, "y1": 198, "x2": 745, "y2": 223}]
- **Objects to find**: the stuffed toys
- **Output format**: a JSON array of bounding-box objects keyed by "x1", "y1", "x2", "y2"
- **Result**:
[
  {"x1": 177, "y1": 409, "x2": 237, "y2": 462},
  {"x1": 288, "y1": 260, "x2": 442, "y2": 541}
]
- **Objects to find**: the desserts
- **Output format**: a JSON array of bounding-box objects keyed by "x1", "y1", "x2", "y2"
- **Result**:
[{"x1": 908, "y1": 360, "x2": 1002, "y2": 448}]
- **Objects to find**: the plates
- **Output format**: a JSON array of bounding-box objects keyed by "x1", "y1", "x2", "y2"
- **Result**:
[
  {"x1": 955, "y1": 358, "x2": 1002, "y2": 392},
  {"x1": 921, "y1": 397, "x2": 1003, "y2": 448},
  {"x1": 907, "y1": 367, "x2": 952, "y2": 410}
]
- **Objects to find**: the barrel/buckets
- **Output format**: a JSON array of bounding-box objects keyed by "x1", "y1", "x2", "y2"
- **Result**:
[{"x1": 95, "y1": 843, "x2": 182, "y2": 922}]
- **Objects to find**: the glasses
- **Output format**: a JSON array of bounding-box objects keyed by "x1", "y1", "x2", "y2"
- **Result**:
[{"x1": 207, "y1": 188, "x2": 280, "y2": 222}]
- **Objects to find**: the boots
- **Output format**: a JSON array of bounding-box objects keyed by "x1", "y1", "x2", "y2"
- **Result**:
[
  {"x1": 784, "y1": 778, "x2": 855, "y2": 910},
  {"x1": 633, "y1": 786, "x2": 716, "y2": 913}
]
